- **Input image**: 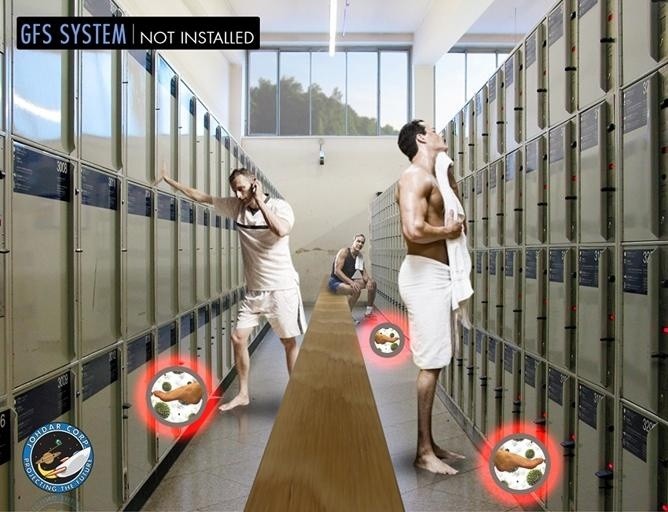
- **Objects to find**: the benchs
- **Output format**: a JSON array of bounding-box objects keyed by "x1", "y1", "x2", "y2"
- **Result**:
[{"x1": 243, "y1": 271, "x2": 407, "y2": 512}]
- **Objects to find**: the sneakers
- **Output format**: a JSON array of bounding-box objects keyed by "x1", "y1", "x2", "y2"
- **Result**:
[
  {"x1": 365, "y1": 314, "x2": 377, "y2": 321},
  {"x1": 353, "y1": 317, "x2": 360, "y2": 326}
]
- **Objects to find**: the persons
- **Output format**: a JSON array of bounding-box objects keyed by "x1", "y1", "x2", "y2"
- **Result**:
[
  {"x1": 327, "y1": 233, "x2": 377, "y2": 326},
  {"x1": 161, "y1": 159, "x2": 308, "y2": 413},
  {"x1": 395, "y1": 119, "x2": 467, "y2": 476}
]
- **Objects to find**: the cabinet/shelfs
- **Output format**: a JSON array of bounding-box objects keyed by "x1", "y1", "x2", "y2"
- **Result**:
[
  {"x1": 0, "y1": 0, "x2": 275, "y2": 512},
  {"x1": 368, "y1": 0, "x2": 668, "y2": 512}
]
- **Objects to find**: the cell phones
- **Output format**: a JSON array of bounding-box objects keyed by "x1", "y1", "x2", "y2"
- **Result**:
[{"x1": 252, "y1": 178, "x2": 258, "y2": 193}]
